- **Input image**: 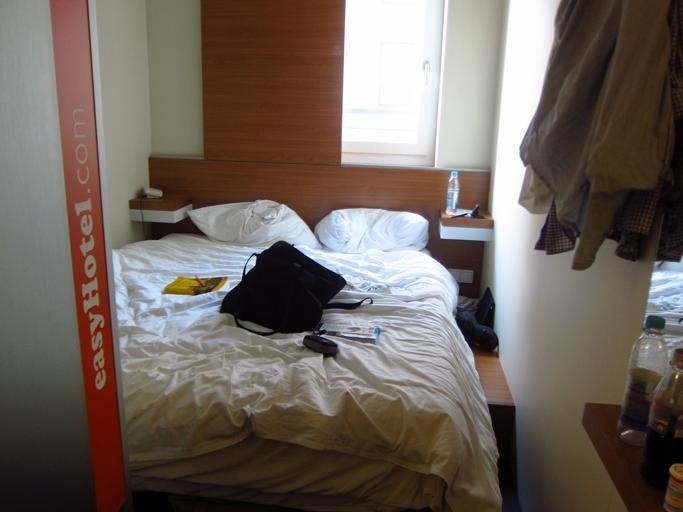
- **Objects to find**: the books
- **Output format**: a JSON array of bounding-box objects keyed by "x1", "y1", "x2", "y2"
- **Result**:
[
  {"x1": 163, "y1": 276, "x2": 230, "y2": 295},
  {"x1": 319, "y1": 324, "x2": 380, "y2": 344}
]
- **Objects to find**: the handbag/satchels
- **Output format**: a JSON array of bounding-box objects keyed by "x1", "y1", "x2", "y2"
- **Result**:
[
  {"x1": 476, "y1": 287, "x2": 495, "y2": 327},
  {"x1": 219, "y1": 241, "x2": 347, "y2": 334}
]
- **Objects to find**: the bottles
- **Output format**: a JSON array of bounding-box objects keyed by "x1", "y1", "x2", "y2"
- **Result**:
[
  {"x1": 444, "y1": 171, "x2": 459, "y2": 217},
  {"x1": 637, "y1": 347, "x2": 682, "y2": 492},
  {"x1": 617, "y1": 312, "x2": 669, "y2": 449}
]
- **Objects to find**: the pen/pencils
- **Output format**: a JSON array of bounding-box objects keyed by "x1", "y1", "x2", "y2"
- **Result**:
[{"x1": 194, "y1": 274, "x2": 205, "y2": 287}]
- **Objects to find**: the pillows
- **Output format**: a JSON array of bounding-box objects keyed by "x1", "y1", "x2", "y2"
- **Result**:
[
  {"x1": 312, "y1": 206, "x2": 431, "y2": 255},
  {"x1": 187, "y1": 197, "x2": 320, "y2": 251}
]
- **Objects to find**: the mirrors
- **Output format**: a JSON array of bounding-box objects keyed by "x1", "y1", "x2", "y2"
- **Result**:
[{"x1": 644, "y1": 114, "x2": 683, "y2": 375}]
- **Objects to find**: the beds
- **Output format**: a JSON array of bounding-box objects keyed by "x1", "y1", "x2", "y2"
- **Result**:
[{"x1": 110, "y1": 231, "x2": 505, "y2": 510}]
